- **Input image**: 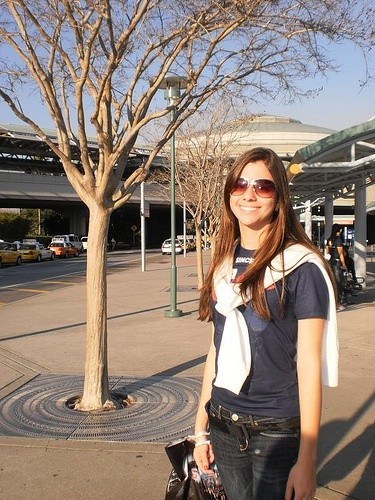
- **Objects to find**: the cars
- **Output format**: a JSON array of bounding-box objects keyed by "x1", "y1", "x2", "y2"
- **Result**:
[
  {"x1": 161, "y1": 238, "x2": 205, "y2": 255},
  {"x1": 0, "y1": 235, "x2": 88, "y2": 267}
]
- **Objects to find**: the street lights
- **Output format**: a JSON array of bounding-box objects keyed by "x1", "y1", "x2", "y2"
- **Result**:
[{"x1": 164, "y1": 87, "x2": 182, "y2": 318}]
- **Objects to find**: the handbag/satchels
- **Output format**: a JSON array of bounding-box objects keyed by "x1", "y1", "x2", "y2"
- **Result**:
[{"x1": 164, "y1": 432, "x2": 228, "y2": 500}]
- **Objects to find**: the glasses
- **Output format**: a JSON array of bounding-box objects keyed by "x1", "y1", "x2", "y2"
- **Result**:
[{"x1": 230, "y1": 178, "x2": 279, "y2": 198}]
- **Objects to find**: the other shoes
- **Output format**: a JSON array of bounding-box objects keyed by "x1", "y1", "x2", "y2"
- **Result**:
[{"x1": 336, "y1": 305, "x2": 345, "y2": 312}]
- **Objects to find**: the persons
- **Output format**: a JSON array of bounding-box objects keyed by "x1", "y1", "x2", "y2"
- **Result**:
[
  {"x1": 323, "y1": 223, "x2": 348, "y2": 312},
  {"x1": 343, "y1": 246, "x2": 356, "y2": 284},
  {"x1": 190, "y1": 146, "x2": 341, "y2": 500}
]
